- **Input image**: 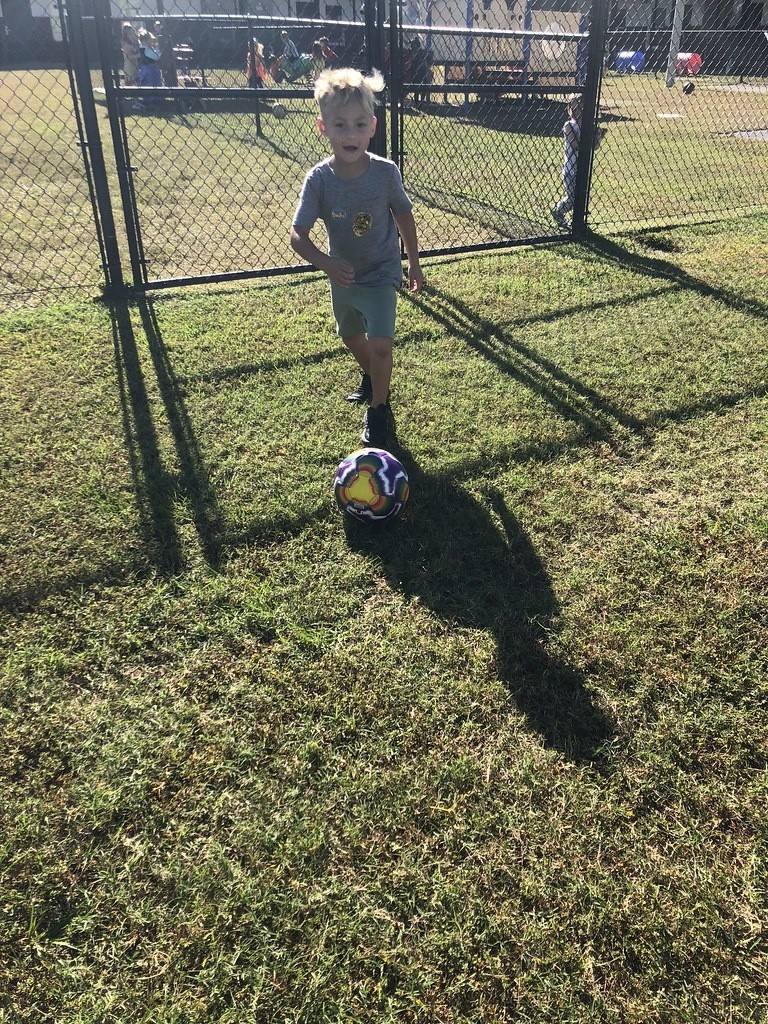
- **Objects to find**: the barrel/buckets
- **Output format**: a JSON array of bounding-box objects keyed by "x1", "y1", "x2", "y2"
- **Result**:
[
  {"x1": 615, "y1": 51, "x2": 644, "y2": 71},
  {"x1": 676, "y1": 53, "x2": 701, "y2": 75}
]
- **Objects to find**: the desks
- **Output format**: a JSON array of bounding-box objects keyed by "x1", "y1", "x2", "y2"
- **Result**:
[{"x1": 172, "y1": 48, "x2": 194, "y2": 75}]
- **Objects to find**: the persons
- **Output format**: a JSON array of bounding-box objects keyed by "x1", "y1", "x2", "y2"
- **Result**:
[
  {"x1": 247, "y1": 30, "x2": 329, "y2": 87},
  {"x1": 289, "y1": 67, "x2": 424, "y2": 445},
  {"x1": 551, "y1": 93, "x2": 609, "y2": 227},
  {"x1": 121, "y1": 20, "x2": 178, "y2": 111}
]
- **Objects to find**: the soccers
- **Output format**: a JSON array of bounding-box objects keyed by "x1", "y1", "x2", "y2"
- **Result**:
[
  {"x1": 272, "y1": 103, "x2": 287, "y2": 118},
  {"x1": 334, "y1": 447, "x2": 410, "y2": 525}
]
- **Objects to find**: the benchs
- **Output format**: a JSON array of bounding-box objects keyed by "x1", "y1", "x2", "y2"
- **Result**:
[{"x1": 177, "y1": 57, "x2": 194, "y2": 61}]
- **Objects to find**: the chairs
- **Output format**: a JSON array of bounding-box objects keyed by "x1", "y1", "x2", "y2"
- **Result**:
[{"x1": 178, "y1": 44, "x2": 191, "y2": 48}]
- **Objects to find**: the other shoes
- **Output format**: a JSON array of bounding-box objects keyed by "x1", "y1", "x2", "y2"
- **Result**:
[
  {"x1": 551, "y1": 207, "x2": 567, "y2": 227},
  {"x1": 133, "y1": 103, "x2": 145, "y2": 110}
]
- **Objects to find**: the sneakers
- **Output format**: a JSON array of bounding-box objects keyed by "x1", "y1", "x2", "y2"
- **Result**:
[
  {"x1": 362, "y1": 404, "x2": 391, "y2": 446},
  {"x1": 346, "y1": 370, "x2": 373, "y2": 405}
]
